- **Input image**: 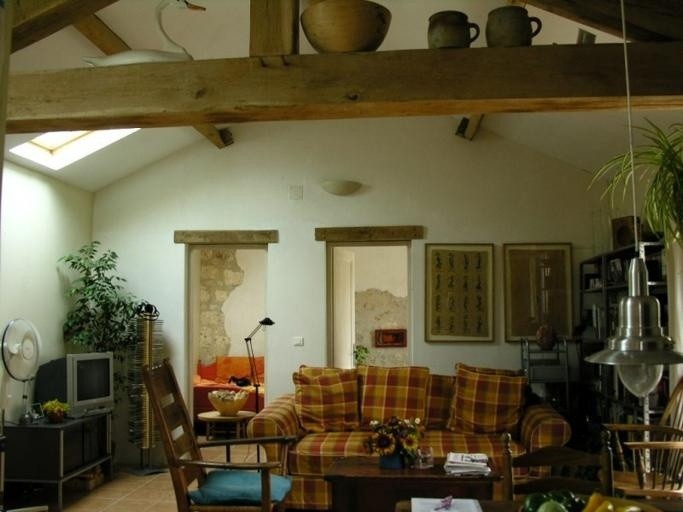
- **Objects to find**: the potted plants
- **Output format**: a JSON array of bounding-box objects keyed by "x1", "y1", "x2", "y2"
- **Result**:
[
  {"x1": 57, "y1": 240, "x2": 149, "y2": 458},
  {"x1": 41, "y1": 398, "x2": 71, "y2": 424}
]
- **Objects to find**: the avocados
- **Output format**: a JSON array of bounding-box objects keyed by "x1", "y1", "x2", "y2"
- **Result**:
[{"x1": 524, "y1": 490, "x2": 587, "y2": 511}]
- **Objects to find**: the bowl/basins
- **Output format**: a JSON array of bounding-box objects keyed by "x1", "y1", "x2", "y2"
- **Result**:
[
  {"x1": 298, "y1": 1, "x2": 392, "y2": 52},
  {"x1": 208, "y1": 388, "x2": 249, "y2": 416}
]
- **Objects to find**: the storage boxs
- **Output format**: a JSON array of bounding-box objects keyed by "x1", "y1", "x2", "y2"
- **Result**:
[{"x1": 612, "y1": 215, "x2": 641, "y2": 249}]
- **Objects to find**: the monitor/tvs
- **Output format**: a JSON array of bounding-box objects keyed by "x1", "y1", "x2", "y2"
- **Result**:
[{"x1": 33, "y1": 350, "x2": 114, "y2": 420}]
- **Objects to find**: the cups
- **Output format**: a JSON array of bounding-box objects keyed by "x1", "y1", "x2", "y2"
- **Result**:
[
  {"x1": 426, "y1": 10, "x2": 480, "y2": 50},
  {"x1": 484, "y1": 5, "x2": 542, "y2": 47}
]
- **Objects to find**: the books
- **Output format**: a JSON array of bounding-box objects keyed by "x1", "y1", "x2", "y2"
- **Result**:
[
  {"x1": 409, "y1": 496, "x2": 483, "y2": 512},
  {"x1": 589, "y1": 300, "x2": 605, "y2": 392},
  {"x1": 441, "y1": 451, "x2": 493, "y2": 480}
]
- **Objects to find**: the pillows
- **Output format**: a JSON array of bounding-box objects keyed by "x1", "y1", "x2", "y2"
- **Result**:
[
  {"x1": 215, "y1": 355, "x2": 264, "y2": 384},
  {"x1": 292, "y1": 363, "x2": 534, "y2": 436}
]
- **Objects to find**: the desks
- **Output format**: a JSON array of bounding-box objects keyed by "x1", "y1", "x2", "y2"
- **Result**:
[{"x1": 395, "y1": 497, "x2": 588, "y2": 512}]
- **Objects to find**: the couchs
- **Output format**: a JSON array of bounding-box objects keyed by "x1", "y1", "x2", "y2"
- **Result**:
[{"x1": 246, "y1": 382, "x2": 572, "y2": 512}]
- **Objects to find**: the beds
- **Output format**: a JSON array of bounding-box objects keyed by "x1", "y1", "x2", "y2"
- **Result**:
[{"x1": 193, "y1": 359, "x2": 264, "y2": 436}]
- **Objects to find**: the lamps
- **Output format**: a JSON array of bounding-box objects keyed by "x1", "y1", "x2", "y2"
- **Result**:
[
  {"x1": 584, "y1": 0, "x2": 683, "y2": 399},
  {"x1": 454, "y1": 112, "x2": 485, "y2": 140},
  {"x1": 244, "y1": 317, "x2": 275, "y2": 472}
]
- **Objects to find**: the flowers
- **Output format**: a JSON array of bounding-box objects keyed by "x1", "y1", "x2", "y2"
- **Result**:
[{"x1": 360, "y1": 414, "x2": 426, "y2": 467}]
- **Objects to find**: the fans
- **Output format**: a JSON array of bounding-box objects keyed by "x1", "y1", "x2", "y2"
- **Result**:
[{"x1": 1, "y1": 318, "x2": 42, "y2": 425}]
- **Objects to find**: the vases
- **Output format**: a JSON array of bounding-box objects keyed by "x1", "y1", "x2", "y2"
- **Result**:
[{"x1": 378, "y1": 444, "x2": 404, "y2": 470}]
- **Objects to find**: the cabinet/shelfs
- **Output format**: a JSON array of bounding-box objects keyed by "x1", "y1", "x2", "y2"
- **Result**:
[
  {"x1": 519, "y1": 337, "x2": 570, "y2": 420},
  {"x1": 579, "y1": 241, "x2": 670, "y2": 455},
  {"x1": 3, "y1": 412, "x2": 112, "y2": 511}
]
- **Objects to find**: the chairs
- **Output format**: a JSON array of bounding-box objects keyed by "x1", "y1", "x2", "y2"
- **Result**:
[
  {"x1": 144, "y1": 357, "x2": 298, "y2": 512},
  {"x1": 600, "y1": 374, "x2": 683, "y2": 500},
  {"x1": 500, "y1": 429, "x2": 614, "y2": 500}
]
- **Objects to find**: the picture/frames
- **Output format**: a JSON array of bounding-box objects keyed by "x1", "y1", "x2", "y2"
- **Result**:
[
  {"x1": 374, "y1": 330, "x2": 408, "y2": 347},
  {"x1": 425, "y1": 242, "x2": 495, "y2": 343},
  {"x1": 502, "y1": 242, "x2": 574, "y2": 342}
]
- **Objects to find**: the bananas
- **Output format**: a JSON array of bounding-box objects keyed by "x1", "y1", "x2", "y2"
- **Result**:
[{"x1": 582, "y1": 492, "x2": 642, "y2": 512}]
